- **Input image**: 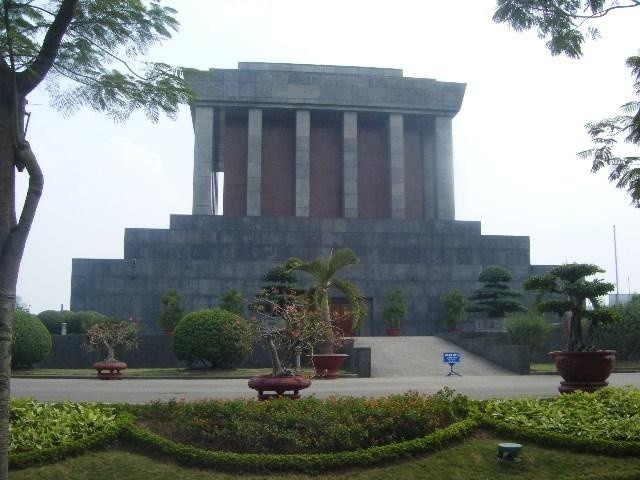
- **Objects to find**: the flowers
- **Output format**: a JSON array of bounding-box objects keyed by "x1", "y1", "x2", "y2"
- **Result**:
[
  {"x1": 85, "y1": 316, "x2": 140, "y2": 361},
  {"x1": 224, "y1": 285, "x2": 353, "y2": 376}
]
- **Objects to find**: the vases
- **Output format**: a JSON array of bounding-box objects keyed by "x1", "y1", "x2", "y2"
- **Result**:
[
  {"x1": 248, "y1": 376, "x2": 311, "y2": 400},
  {"x1": 94, "y1": 361, "x2": 128, "y2": 378}
]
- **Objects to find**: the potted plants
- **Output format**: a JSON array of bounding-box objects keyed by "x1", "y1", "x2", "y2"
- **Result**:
[
  {"x1": 522, "y1": 262, "x2": 624, "y2": 393},
  {"x1": 441, "y1": 287, "x2": 471, "y2": 333},
  {"x1": 158, "y1": 288, "x2": 186, "y2": 335},
  {"x1": 383, "y1": 287, "x2": 407, "y2": 336},
  {"x1": 283, "y1": 247, "x2": 368, "y2": 378}
]
